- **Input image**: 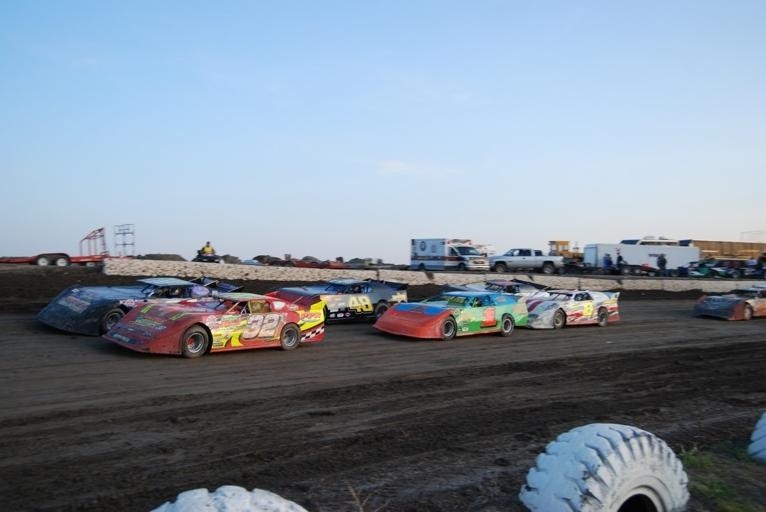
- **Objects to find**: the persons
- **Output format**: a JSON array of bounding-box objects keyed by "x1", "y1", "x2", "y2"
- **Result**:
[
  {"x1": 602, "y1": 251, "x2": 627, "y2": 276},
  {"x1": 347, "y1": 285, "x2": 361, "y2": 293},
  {"x1": 154, "y1": 287, "x2": 182, "y2": 298},
  {"x1": 658, "y1": 253, "x2": 668, "y2": 270},
  {"x1": 201, "y1": 241, "x2": 215, "y2": 255}
]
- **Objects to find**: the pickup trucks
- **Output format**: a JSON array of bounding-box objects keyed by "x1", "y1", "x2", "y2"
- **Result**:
[{"x1": 488, "y1": 247, "x2": 565, "y2": 274}]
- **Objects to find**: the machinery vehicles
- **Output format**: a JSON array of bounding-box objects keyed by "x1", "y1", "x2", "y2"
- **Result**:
[{"x1": 547, "y1": 241, "x2": 584, "y2": 271}]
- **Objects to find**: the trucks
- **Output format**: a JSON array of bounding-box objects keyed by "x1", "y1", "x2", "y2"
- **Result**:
[{"x1": 409, "y1": 238, "x2": 491, "y2": 272}]
